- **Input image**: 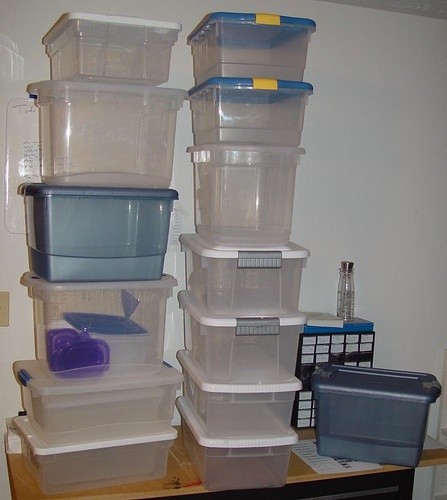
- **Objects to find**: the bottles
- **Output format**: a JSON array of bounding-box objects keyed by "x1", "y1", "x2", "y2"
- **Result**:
[{"x1": 336, "y1": 260, "x2": 356, "y2": 323}]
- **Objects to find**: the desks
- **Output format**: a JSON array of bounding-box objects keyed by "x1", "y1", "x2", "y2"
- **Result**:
[{"x1": 4, "y1": 425, "x2": 447, "y2": 499}]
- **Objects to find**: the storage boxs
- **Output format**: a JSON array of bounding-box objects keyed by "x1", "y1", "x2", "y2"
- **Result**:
[{"x1": 12, "y1": 11, "x2": 442, "y2": 489}]
